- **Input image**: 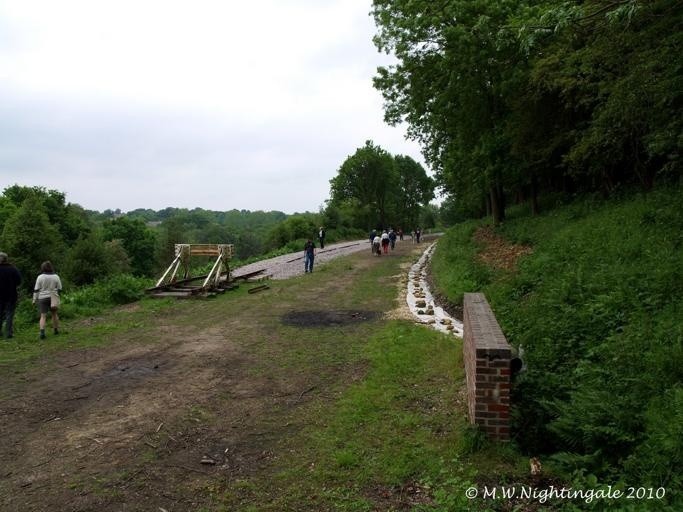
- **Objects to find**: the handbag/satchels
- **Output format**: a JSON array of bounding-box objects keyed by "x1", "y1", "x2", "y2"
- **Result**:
[{"x1": 50, "y1": 294, "x2": 59, "y2": 310}]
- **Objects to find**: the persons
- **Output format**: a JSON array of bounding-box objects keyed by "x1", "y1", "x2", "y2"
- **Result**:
[
  {"x1": 369, "y1": 221, "x2": 423, "y2": 258},
  {"x1": 0, "y1": 253, "x2": 22, "y2": 341},
  {"x1": 303, "y1": 239, "x2": 318, "y2": 274},
  {"x1": 316, "y1": 224, "x2": 326, "y2": 251},
  {"x1": 30, "y1": 261, "x2": 64, "y2": 340}
]
